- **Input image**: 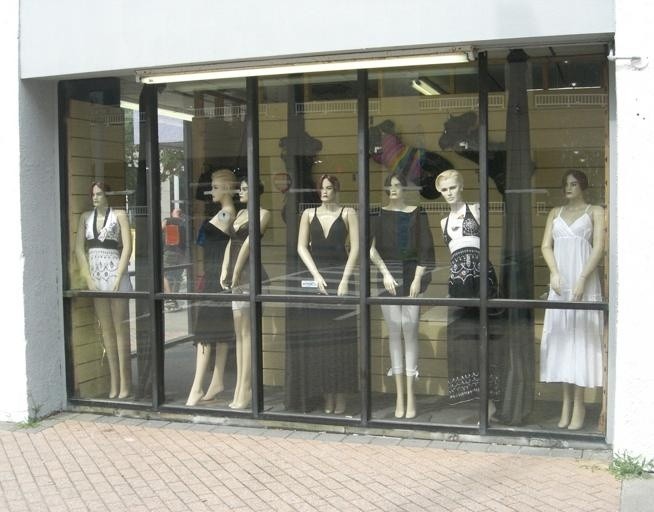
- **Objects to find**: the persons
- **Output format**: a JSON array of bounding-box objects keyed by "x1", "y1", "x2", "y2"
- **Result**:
[
  {"x1": 433, "y1": 170, "x2": 506, "y2": 424},
  {"x1": 439, "y1": 111, "x2": 538, "y2": 200},
  {"x1": 220, "y1": 175, "x2": 270, "y2": 413},
  {"x1": 74, "y1": 182, "x2": 137, "y2": 399},
  {"x1": 363, "y1": 121, "x2": 452, "y2": 198},
  {"x1": 274, "y1": 127, "x2": 323, "y2": 223},
  {"x1": 160, "y1": 208, "x2": 185, "y2": 315},
  {"x1": 187, "y1": 168, "x2": 237, "y2": 406},
  {"x1": 540, "y1": 169, "x2": 605, "y2": 435},
  {"x1": 298, "y1": 173, "x2": 359, "y2": 415},
  {"x1": 367, "y1": 173, "x2": 437, "y2": 421}
]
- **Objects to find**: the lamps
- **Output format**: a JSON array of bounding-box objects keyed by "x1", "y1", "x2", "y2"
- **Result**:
[
  {"x1": 137, "y1": 43, "x2": 477, "y2": 84},
  {"x1": 408, "y1": 74, "x2": 448, "y2": 95}
]
